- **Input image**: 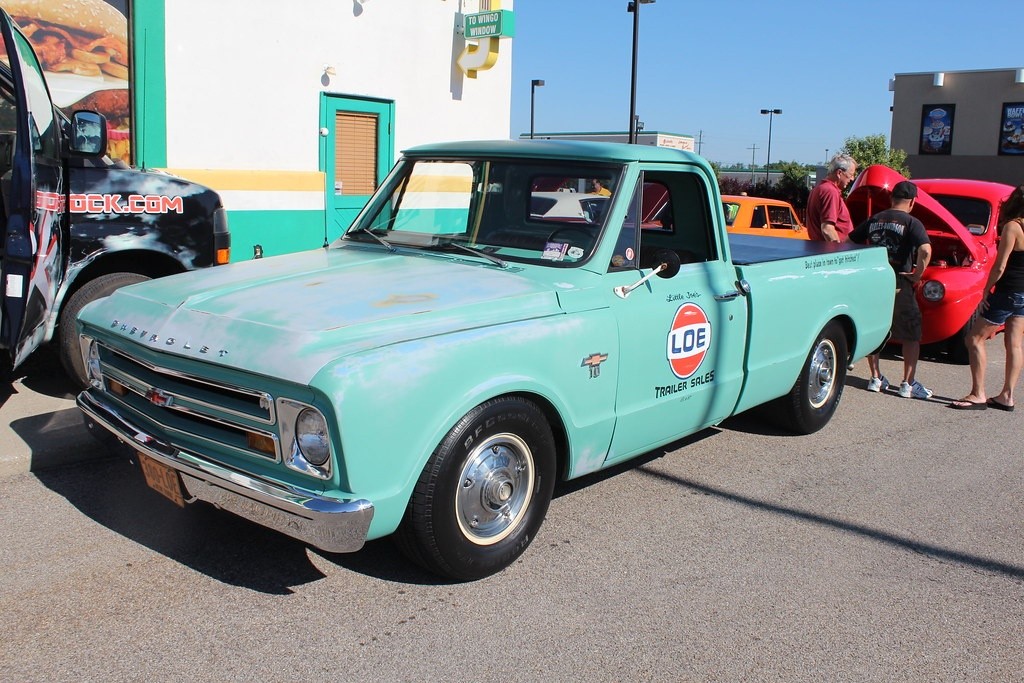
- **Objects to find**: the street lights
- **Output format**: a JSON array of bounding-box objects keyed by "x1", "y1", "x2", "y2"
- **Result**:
[
  {"x1": 634, "y1": 114, "x2": 640, "y2": 144},
  {"x1": 625, "y1": 0, "x2": 657, "y2": 145},
  {"x1": 760, "y1": 109, "x2": 783, "y2": 188},
  {"x1": 825, "y1": 149, "x2": 829, "y2": 166}
]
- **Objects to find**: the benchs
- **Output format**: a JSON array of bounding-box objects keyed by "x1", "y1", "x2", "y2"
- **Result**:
[{"x1": 487, "y1": 229, "x2": 709, "y2": 267}]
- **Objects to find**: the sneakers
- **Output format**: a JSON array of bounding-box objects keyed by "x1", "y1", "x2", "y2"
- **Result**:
[
  {"x1": 898, "y1": 381, "x2": 933, "y2": 399},
  {"x1": 867, "y1": 376, "x2": 889, "y2": 392}
]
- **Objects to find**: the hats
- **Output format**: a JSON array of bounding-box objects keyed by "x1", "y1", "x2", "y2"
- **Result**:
[{"x1": 891, "y1": 181, "x2": 918, "y2": 199}]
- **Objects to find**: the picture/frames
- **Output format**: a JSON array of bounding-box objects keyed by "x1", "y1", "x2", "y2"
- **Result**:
[
  {"x1": 998, "y1": 102, "x2": 1024, "y2": 156},
  {"x1": 918, "y1": 104, "x2": 956, "y2": 155}
]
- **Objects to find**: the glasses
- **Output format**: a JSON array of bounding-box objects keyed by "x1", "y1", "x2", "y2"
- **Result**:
[{"x1": 842, "y1": 171, "x2": 858, "y2": 177}]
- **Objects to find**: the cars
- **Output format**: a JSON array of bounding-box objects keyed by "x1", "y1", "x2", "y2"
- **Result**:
[
  {"x1": 842, "y1": 163, "x2": 1024, "y2": 347},
  {"x1": 543, "y1": 183, "x2": 674, "y2": 231},
  {"x1": 528, "y1": 79, "x2": 545, "y2": 140},
  {"x1": 720, "y1": 193, "x2": 812, "y2": 241},
  {"x1": 530, "y1": 191, "x2": 610, "y2": 223}
]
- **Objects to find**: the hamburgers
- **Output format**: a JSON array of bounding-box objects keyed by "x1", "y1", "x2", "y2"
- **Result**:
[{"x1": 0, "y1": 0, "x2": 129, "y2": 166}]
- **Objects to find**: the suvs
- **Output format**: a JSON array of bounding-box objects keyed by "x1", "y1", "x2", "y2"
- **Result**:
[{"x1": 1, "y1": 7, "x2": 232, "y2": 400}]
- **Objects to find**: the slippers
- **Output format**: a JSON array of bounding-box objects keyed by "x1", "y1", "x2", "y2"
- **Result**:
[
  {"x1": 952, "y1": 398, "x2": 988, "y2": 410},
  {"x1": 987, "y1": 398, "x2": 1014, "y2": 411}
]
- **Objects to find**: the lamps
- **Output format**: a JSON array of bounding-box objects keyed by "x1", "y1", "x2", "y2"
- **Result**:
[
  {"x1": 889, "y1": 79, "x2": 895, "y2": 91},
  {"x1": 933, "y1": 73, "x2": 944, "y2": 87},
  {"x1": 325, "y1": 66, "x2": 336, "y2": 76},
  {"x1": 1015, "y1": 69, "x2": 1024, "y2": 83}
]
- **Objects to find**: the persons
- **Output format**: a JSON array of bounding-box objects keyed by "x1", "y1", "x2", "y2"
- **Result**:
[
  {"x1": 724, "y1": 192, "x2": 746, "y2": 222},
  {"x1": 588, "y1": 179, "x2": 612, "y2": 196},
  {"x1": 806, "y1": 154, "x2": 857, "y2": 243},
  {"x1": 846, "y1": 181, "x2": 933, "y2": 399},
  {"x1": 950, "y1": 192, "x2": 1024, "y2": 411}
]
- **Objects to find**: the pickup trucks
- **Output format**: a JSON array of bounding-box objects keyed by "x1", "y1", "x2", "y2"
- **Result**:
[{"x1": 71, "y1": 137, "x2": 901, "y2": 581}]
